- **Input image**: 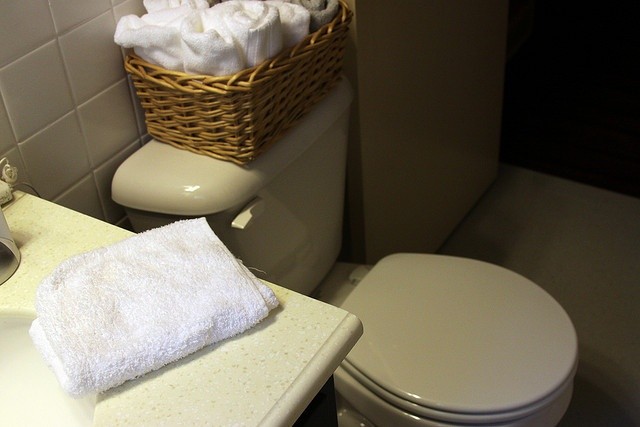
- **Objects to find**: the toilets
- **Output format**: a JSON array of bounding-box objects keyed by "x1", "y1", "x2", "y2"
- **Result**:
[{"x1": 111, "y1": 75, "x2": 580, "y2": 427}]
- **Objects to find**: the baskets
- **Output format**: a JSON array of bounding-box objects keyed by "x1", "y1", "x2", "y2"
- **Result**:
[{"x1": 122, "y1": 1, "x2": 354, "y2": 167}]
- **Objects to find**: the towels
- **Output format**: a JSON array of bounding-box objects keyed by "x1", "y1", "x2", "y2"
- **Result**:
[
  {"x1": 114, "y1": 1, "x2": 340, "y2": 80},
  {"x1": 34, "y1": 212, "x2": 276, "y2": 397}
]
